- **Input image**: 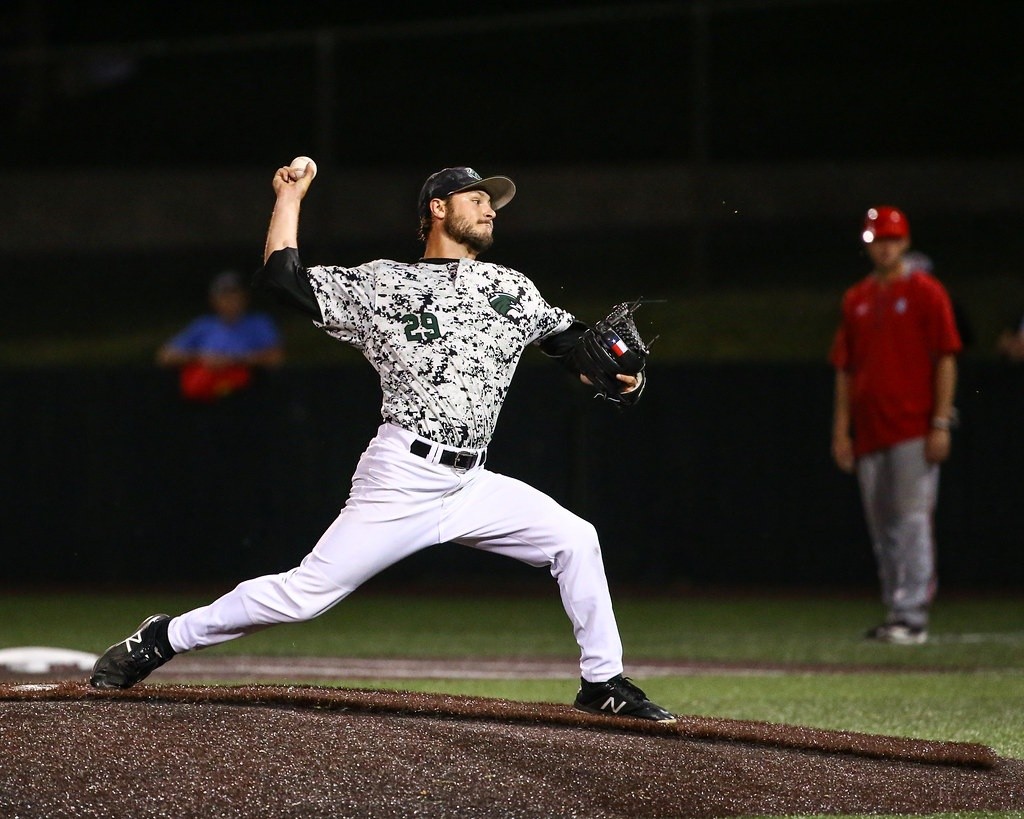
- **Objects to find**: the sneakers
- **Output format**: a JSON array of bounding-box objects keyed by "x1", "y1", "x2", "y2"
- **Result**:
[
  {"x1": 87, "y1": 612, "x2": 172, "y2": 692},
  {"x1": 573, "y1": 676, "x2": 677, "y2": 724}
]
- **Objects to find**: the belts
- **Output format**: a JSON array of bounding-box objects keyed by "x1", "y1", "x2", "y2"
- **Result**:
[{"x1": 410, "y1": 439, "x2": 486, "y2": 471}]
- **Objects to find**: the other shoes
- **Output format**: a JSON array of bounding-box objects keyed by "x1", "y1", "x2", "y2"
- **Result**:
[{"x1": 866, "y1": 620, "x2": 929, "y2": 646}]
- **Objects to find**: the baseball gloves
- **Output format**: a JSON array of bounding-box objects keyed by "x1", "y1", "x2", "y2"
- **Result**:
[{"x1": 574, "y1": 294, "x2": 660, "y2": 413}]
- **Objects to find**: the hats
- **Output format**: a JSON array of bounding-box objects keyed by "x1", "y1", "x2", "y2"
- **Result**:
[
  {"x1": 863, "y1": 205, "x2": 911, "y2": 240},
  {"x1": 416, "y1": 166, "x2": 517, "y2": 212}
]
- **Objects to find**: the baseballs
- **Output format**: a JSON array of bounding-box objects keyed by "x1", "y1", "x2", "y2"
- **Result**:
[{"x1": 288, "y1": 155, "x2": 318, "y2": 183}]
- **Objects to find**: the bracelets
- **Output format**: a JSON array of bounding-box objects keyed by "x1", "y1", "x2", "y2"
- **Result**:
[{"x1": 929, "y1": 416, "x2": 951, "y2": 430}]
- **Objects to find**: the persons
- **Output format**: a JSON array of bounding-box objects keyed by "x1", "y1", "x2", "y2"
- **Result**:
[
  {"x1": 982, "y1": 310, "x2": 1024, "y2": 361},
  {"x1": 154, "y1": 269, "x2": 282, "y2": 367},
  {"x1": 834, "y1": 207, "x2": 962, "y2": 646},
  {"x1": 90, "y1": 163, "x2": 676, "y2": 723}
]
